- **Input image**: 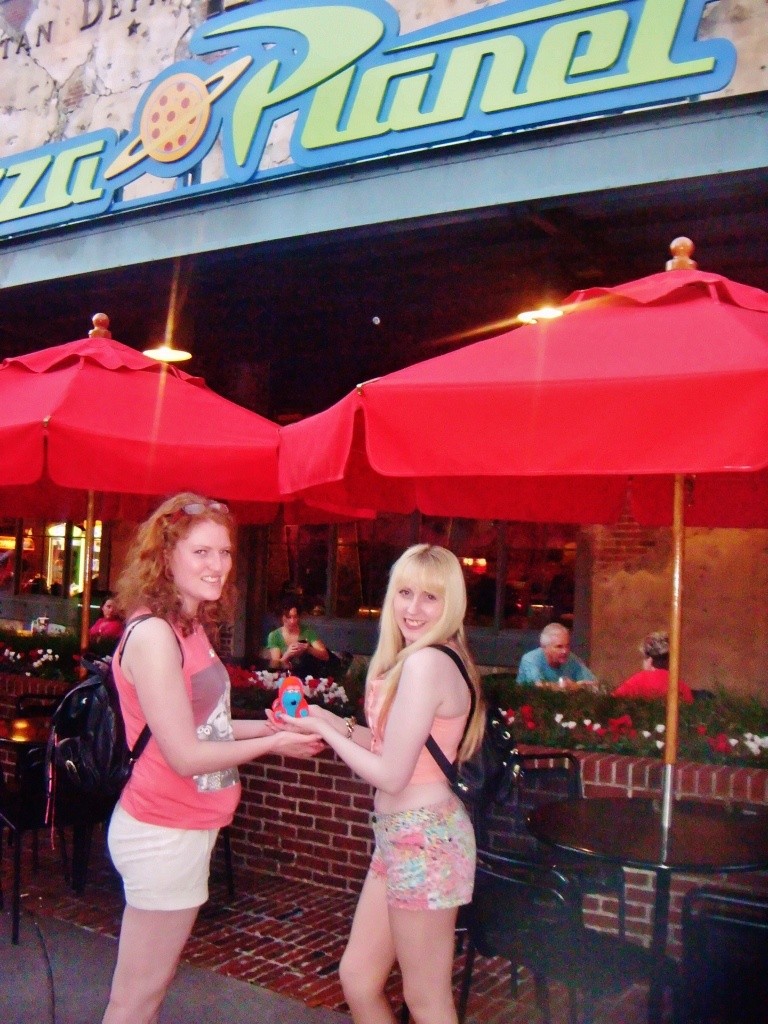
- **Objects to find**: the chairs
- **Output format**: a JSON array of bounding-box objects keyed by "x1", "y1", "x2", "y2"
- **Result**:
[
  {"x1": 9, "y1": 692, "x2": 79, "y2": 868},
  {"x1": 401, "y1": 749, "x2": 768, "y2": 1024}
]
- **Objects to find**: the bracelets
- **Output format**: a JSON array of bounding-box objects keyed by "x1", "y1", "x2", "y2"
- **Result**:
[{"x1": 344, "y1": 717, "x2": 353, "y2": 741}]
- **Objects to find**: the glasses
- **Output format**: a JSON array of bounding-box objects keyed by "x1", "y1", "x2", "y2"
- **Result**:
[{"x1": 182, "y1": 502, "x2": 229, "y2": 516}]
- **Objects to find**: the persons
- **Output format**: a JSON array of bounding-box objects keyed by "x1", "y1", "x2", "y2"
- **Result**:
[
  {"x1": 87, "y1": 594, "x2": 691, "y2": 706},
  {"x1": 263, "y1": 543, "x2": 481, "y2": 1023},
  {"x1": 99, "y1": 494, "x2": 328, "y2": 1023}
]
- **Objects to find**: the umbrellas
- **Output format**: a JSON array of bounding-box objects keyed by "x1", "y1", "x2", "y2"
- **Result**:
[
  {"x1": 0, "y1": 314, "x2": 288, "y2": 684},
  {"x1": 277, "y1": 235, "x2": 767, "y2": 865}
]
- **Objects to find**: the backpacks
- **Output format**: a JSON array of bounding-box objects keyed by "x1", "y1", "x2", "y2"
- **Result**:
[
  {"x1": 46, "y1": 616, "x2": 186, "y2": 805},
  {"x1": 421, "y1": 644, "x2": 522, "y2": 804}
]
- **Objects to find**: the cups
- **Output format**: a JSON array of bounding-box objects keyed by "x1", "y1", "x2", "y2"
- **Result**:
[
  {"x1": 39, "y1": 617, "x2": 50, "y2": 636},
  {"x1": 560, "y1": 676, "x2": 573, "y2": 693}
]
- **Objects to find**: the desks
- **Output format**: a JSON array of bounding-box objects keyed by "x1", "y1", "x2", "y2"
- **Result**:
[
  {"x1": 525, "y1": 793, "x2": 767, "y2": 1022},
  {"x1": 0, "y1": 714, "x2": 93, "y2": 945}
]
